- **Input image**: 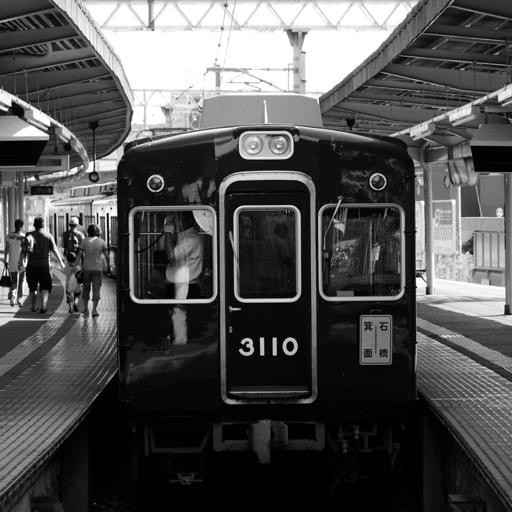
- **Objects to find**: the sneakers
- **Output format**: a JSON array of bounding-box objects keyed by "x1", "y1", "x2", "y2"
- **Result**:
[
  {"x1": 8, "y1": 293, "x2": 24, "y2": 310},
  {"x1": 29, "y1": 306, "x2": 47, "y2": 313},
  {"x1": 68, "y1": 303, "x2": 99, "y2": 316}
]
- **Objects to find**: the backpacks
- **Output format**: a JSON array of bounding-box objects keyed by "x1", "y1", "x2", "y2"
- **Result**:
[
  {"x1": 67, "y1": 272, "x2": 79, "y2": 293},
  {"x1": 65, "y1": 233, "x2": 79, "y2": 253}
]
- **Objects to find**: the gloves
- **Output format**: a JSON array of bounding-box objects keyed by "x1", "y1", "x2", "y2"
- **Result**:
[{"x1": 163, "y1": 215, "x2": 175, "y2": 235}]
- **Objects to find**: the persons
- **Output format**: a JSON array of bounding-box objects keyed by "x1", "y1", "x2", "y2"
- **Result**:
[
  {"x1": 67, "y1": 224, "x2": 112, "y2": 318},
  {"x1": 60, "y1": 215, "x2": 86, "y2": 313},
  {"x1": 156, "y1": 212, "x2": 207, "y2": 300},
  {"x1": 5, "y1": 218, "x2": 29, "y2": 308},
  {"x1": 254, "y1": 221, "x2": 294, "y2": 295},
  {"x1": 16, "y1": 216, "x2": 67, "y2": 315}
]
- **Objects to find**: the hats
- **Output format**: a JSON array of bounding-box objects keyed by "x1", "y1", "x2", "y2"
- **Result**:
[
  {"x1": 68, "y1": 216, "x2": 80, "y2": 227},
  {"x1": 33, "y1": 216, "x2": 44, "y2": 229}
]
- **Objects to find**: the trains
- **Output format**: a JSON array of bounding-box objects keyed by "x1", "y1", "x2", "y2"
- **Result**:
[{"x1": 46, "y1": 88, "x2": 421, "y2": 489}]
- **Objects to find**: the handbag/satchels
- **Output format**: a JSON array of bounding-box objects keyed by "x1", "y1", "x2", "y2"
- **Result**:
[
  {"x1": 75, "y1": 269, "x2": 83, "y2": 282},
  {"x1": 0, "y1": 265, "x2": 11, "y2": 287}
]
